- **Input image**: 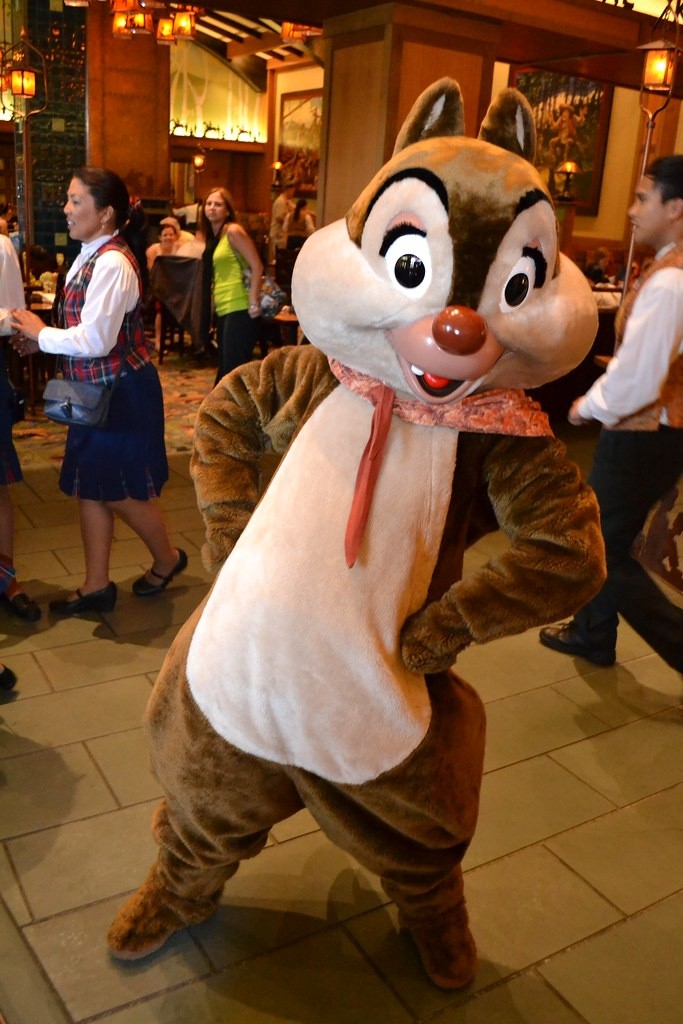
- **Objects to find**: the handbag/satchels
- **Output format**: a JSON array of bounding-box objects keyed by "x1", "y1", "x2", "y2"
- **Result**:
[
  {"x1": 42, "y1": 378, "x2": 113, "y2": 430},
  {"x1": 226, "y1": 227, "x2": 288, "y2": 318}
]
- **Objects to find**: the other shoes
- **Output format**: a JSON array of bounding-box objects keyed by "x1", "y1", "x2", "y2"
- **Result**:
[
  {"x1": 0, "y1": 665, "x2": 17, "y2": 691},
  {"x1": 1, "y1": 588, "x2": 42, "y2": 622}
]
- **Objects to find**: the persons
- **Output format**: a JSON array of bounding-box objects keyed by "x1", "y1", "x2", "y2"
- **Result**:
[
  {"x1": 585, "y1": 246, "x2": 612, "y2": 284},
  {"x1": 0, "y1": 165, "x2": 187, "y2": 694},
  {"x1": 538, "y1": 155, "x2": 683, "y2": 671},
  {"x1": 146, "y1": 184, "x2": 316, "y2": 394}
]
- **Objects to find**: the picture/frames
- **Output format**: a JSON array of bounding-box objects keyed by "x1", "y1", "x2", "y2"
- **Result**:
[
  {"x1": 276, "y1": 87, "x2": 324, "y2": 200},
  {"x1": 510, "y1": 66, "x2": 616, "y2": 219}
]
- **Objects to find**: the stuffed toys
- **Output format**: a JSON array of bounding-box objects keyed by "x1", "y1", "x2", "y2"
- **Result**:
[{"x1": 108, "y1": 76, "x2": 606, "y2": 991}]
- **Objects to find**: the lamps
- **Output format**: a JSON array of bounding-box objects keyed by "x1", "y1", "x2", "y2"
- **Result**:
[
  {"x1": 556, "y1": 161, "x2": 584, "y2": 203},
  {"x1": 621, "y1": 0, "x2": 683, "y2": 306},
  {"x1": 0, "y1": 25, "x2": 49, "y2": 423},
  {"x1": 109, "y1": 0, "x2": 196, "y2": 47},
  {"x1": 192, "y1": 143, "x2": 206, "y2": 174}
]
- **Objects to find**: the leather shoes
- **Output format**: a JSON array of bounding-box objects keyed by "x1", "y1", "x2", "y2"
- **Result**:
[{"x1": 539, "y1": 618, "x2": 614, "y2": 667}]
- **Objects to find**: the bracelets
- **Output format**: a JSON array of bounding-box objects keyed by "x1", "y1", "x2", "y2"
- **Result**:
[{"x1": 251, "y1": 303, "x2": 257, "y2": 309}]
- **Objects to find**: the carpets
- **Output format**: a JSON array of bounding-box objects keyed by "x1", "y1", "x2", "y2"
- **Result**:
[{"x1": 10, "y1": 340, "x2": 223, "y2": 473}]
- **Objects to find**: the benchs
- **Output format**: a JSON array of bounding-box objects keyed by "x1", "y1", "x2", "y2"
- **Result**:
[{"x1": 575, "y1": 248, "x2": 657, "y2": 276}]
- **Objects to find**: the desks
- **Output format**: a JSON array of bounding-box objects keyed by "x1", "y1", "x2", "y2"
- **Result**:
[
  {"x1": 21, "y1": 293, "x2": 57, "y2": 311},
  {"x1": 587, "y1": 274, "x2": 625, "y2": 313}
]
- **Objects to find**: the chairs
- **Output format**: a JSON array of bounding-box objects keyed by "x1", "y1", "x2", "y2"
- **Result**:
[
  {"x1": 272, "y1": 246, "x2": 303, "y2": 285},
  {"x1": 14, "y1": 244, "x2": 57, "y2": 283},
  {"x1": 154, "y1": 255, "x2": 218, "y2": 370}
]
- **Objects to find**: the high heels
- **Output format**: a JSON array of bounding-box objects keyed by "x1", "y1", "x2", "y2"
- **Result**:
[
  {"x1": 48, "y1": 580, "x2": 117, "y2": 612},
  {"x1": 132, "y1": 547, "x2": 189, "y2": 596}
]
